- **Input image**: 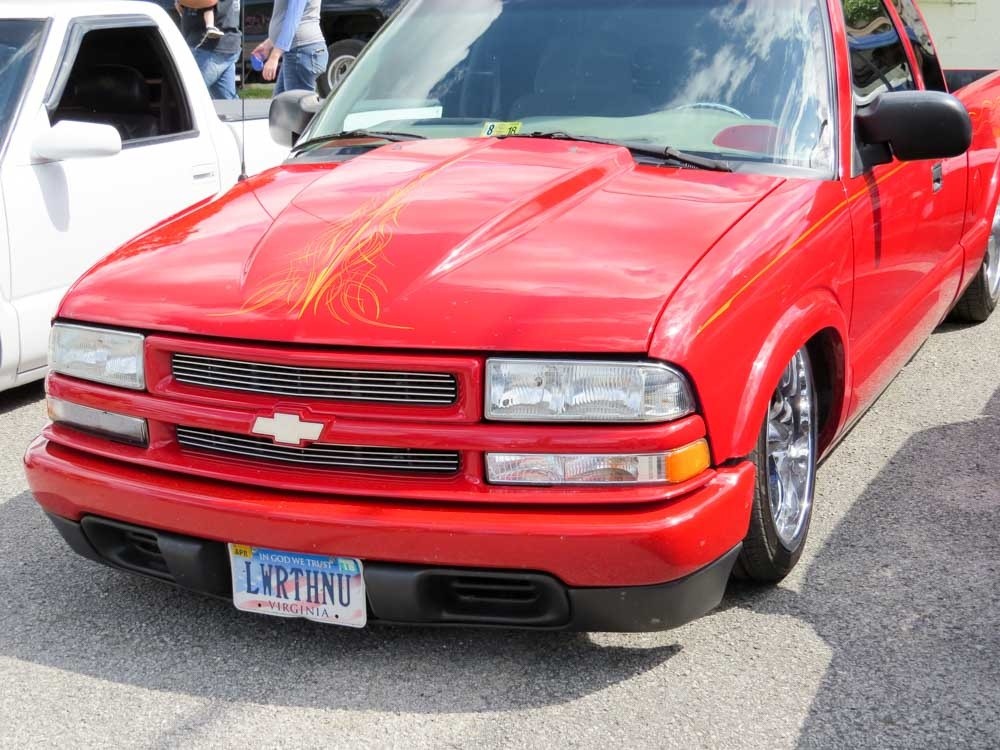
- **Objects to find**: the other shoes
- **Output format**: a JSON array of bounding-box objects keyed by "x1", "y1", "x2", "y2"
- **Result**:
[{"x1": 206, "y1": 28, "x2": 223, "y2": 39}]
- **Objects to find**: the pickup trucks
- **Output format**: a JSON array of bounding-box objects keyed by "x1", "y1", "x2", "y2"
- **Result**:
[
  {"x1": 16, "y1": 0, "x2": 1000, "y2": 637},
  {"x1": 1, "y1": 0, "x2": 445, "y2": 396}
]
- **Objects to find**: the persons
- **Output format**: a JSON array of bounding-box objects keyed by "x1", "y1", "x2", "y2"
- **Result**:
[
  {"x1": 174, "y1": 0, "x2": 241, "y2": 100},
  {"x1": 251, "y1": 0, "x2": 329, "y2": 95}
]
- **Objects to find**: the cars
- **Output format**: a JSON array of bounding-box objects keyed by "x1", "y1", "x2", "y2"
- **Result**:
[{"x1": 143, "y1": 0, "x2": 405, "y2": 100}]
